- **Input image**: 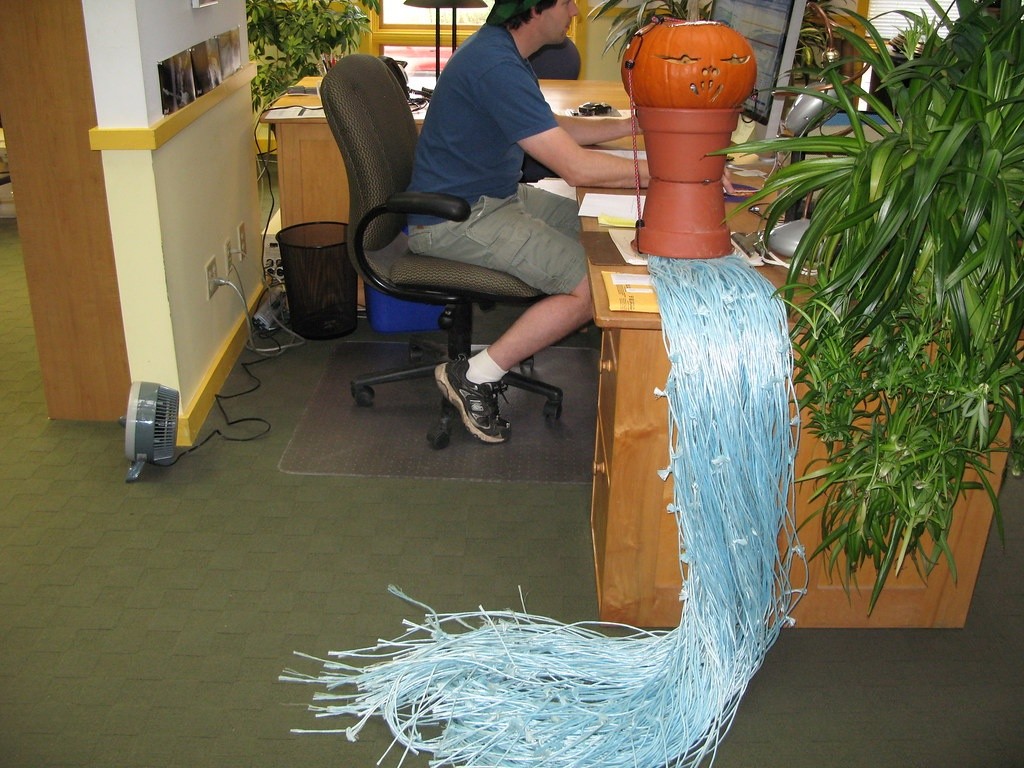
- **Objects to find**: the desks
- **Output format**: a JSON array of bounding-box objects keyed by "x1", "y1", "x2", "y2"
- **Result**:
[
  {"x1": 867, "y1": 46, "x2": 922, "y2": 116},
  {"x1": 261, "y1": 79, "x2": 1024, "y2": 627}
]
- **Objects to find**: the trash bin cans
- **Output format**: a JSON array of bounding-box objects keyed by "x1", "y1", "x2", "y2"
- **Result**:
[{"x1": 275, "y1": 220, "x2": 360, "y2": 342}]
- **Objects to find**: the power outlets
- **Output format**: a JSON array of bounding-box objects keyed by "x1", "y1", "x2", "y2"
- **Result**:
[
  {"x1": 225, "y1": 238, "x2": 233, "y2": 275},
  {"x1": 237, "y1": 221, "x2": 247, "y2": 261},
  {"x1": 205, "y1": 255, "x2": 219, "y2": 299}
]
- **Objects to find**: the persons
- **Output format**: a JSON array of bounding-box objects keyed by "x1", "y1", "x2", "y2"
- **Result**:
[{"x1": 402, "y1": 0, "x2": 735, "y2": 443}]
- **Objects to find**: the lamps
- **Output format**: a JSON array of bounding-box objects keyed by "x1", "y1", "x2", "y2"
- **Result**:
[
  {"x1": 404, "y1": 0, "x2": 488, "y2": 98},
  {"x1": 768, "y1": 81, "x2": 840, "y2": 260}
]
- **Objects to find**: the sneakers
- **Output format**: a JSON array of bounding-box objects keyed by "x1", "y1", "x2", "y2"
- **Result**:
[{"x1": 434, "y1": 353, "x2": 512, "y2": 443}]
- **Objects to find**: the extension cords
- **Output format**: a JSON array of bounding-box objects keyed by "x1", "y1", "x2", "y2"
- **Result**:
[{"x1": 256, "y1": 288, "x2": 285, "y2": 329}]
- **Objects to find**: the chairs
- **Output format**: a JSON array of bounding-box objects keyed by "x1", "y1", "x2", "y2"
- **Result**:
[
  {"x1": 529, "y1": 37, "x2": 580, "y2": 80},
  {"x1": 320, "y1": 54, "x2": 563, "y2": 452}
]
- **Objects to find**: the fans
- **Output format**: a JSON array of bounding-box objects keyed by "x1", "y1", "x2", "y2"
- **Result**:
[{"x1": 118, "y1": 381, "x2": 180, "y2": 483}]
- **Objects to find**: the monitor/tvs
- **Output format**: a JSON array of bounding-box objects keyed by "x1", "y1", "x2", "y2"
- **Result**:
[{"x1": 708, "y1": 0, "x2": 806, "y2": 165}]
